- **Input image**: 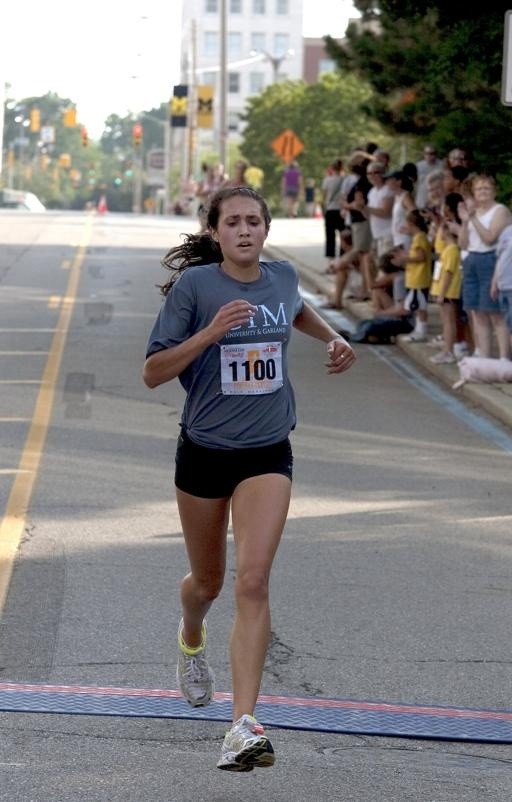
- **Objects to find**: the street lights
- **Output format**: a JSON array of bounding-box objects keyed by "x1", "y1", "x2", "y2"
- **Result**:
[{"x1": 14, "y1": 114, "x2": 30, "y2": 189}]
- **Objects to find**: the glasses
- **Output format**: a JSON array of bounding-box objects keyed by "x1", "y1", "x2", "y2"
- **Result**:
[
  {"x1": 366, "y1": 171, "x2": 381, "y2": 174},
  {"x1": 448, "y1": 156, "x2": 465, "y2": 162},
  {"x1": 425, "y1": 151, "x2": 435, "y2": 155}
]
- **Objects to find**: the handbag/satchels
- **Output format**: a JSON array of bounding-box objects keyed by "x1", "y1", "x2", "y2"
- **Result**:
[
  {"x1": 351, "y1": 318, "x2": 414, "y2": 344},
  {"x1": 458, "y1": 357, "x2": 512, "y2": 384}
]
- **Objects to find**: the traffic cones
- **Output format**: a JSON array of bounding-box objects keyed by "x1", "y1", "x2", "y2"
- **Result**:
[{"x1": 95, "y1": 194, "x2": 107, "y2": 215}]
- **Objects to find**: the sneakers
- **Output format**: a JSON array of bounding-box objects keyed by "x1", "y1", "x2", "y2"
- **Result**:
[
  {"x1": 429, "y1": 352, "x2": 456, "y2": 364},
  {"x1": 216, "y1": 714, "x2": 275, "y2": 772},
  {"x1": 403, "y1": 332, "x2": 426, "y2": 342},
  {"x1": 454, "y1": 348, "x2": 469, "y2": 358},
  {"x1": 178, "y1": 617, "x2": 214, "y2": 709},
  {"x1": 433, "y1": 335, "x2": 445, "y2": 344}
]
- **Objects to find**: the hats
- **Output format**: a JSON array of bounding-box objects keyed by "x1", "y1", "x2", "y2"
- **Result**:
[{"x1": 382, "y1": 170, "x2": 406, "y2": 179}]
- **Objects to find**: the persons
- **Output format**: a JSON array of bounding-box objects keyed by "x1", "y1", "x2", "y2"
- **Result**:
[
  {"x1": 141, "y1": 184, "x2": 360, "y2": 771},
  {"x1": 316, "y1": 142, "x2": 512, "y2": 379},
  {"x1": 140, "y1": 159, "x2": 317, "y2": 233}
]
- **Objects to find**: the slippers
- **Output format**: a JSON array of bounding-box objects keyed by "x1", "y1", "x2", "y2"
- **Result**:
[{"x1": 320, "y1": 302, "x2": 342, "y2": 310}]
